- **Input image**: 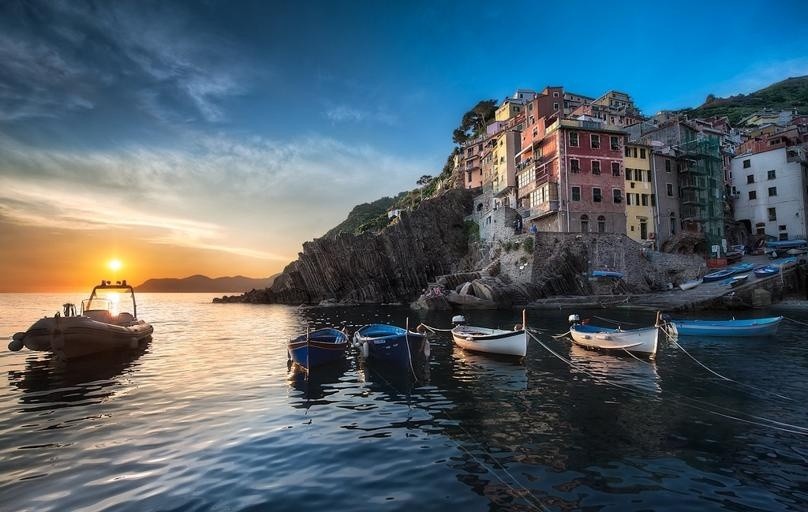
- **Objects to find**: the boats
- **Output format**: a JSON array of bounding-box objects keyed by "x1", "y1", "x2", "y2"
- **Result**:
[
  {"x1": 702, "y1": 250, "x2": 802, "y2": 289},
  {"x1": 8, "y1": 280, "x2": 154, "y2": 358},
  {"x1": 355, "y1": 323, "x2": 427, "y2": 374},
  {"x1": 451, "y1": 315, "x2": 529, "y2": 358},
  {"x1": 569, "y1": 314, "x2": 661, "y2": 360},
  {"x1": 663, "y1": 313, "x2": 784, "y2": 339},
  {"x1": 288, "y1": 326, "x2": 349, "y2": 372}
]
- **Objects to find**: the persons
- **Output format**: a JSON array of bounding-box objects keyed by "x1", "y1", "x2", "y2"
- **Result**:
[
  {"x1": 422, "y1": 287, "x2": 426, "y2": 294},
  {"x1": 435, "y1": 288, "x2": 440, "y2": 296},
  {"x1": 513, "y1": 219, "x2": 522, "y2": 234},
  {"x1": 527, "y1": 223, "x2": 537, "y2": 234}
]
